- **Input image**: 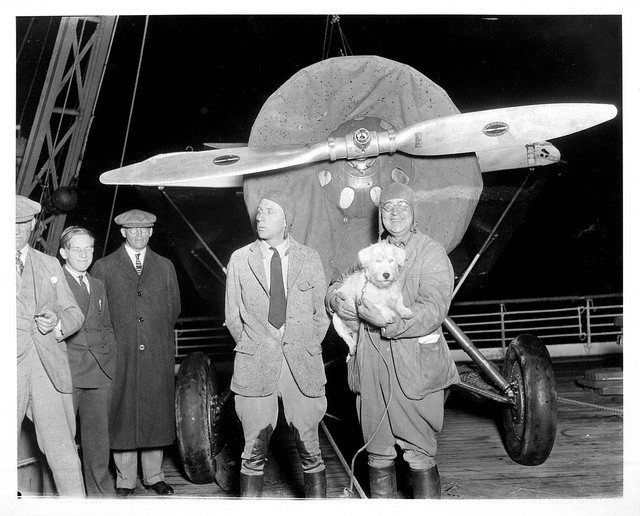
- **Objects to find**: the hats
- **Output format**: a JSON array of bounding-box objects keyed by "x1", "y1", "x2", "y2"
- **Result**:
[
  {"x1": 260, "y1": 190, "x2": 295, "y2": 239},
  {"x1": 114, "y1": 210, "x2": 156, "y2": 228},
  {"x1": 16, "y1": 195, "x2": 41, "y2": 223},
  {"x1": 378, "y1": 182, "x2": 418, "y2": 242}
]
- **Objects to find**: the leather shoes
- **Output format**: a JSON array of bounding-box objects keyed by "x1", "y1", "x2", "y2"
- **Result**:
[
  {"x1": 144, "y1": 481, "x2": 174, "y2": 495},
  {"x1": 116, "y1": 488, "x2": 134, "y2": 496}
]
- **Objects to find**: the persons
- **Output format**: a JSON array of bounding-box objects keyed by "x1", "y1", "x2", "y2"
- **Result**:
[
  {"x1": 224, "y1": 190, "x2": 332, "y2": 498},
  {"x1": 58, "y1": 225, "x2": 118, "y2": 497},
  {"x1": 15, "y1": 195, "x2": 85, "y2": 497},
  {"x1": 326, "y1": 183, "x2": 461, "y2": 499},
  {"x1": 90, "y1": 209, "x2": 182, "y2": 496}
]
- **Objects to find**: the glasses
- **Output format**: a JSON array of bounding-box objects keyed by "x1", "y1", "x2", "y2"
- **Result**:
[
  {"x1": 127, "y1": 228, "x2": 149, "y2": 234},
  {"x1": 381, "y1": 201, "x2": 412, "y2": 212}
]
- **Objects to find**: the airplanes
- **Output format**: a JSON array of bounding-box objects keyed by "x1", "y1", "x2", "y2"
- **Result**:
[{"x1": 99, "y1": 55, "x2": 618, "y2": 483}]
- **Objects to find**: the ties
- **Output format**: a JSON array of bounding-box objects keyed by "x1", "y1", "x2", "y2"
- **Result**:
[
  {"x1": 268, "y1": 247, "x2": 286, "y2": 329},
  {"x1": 16, "y1": 250, "x2": 24, "y2": 276},
  {"x1": 77, "y1": 275, "x2": 90, "y2": 299},
  {"x1": 135, "y1": 254, "x2": 143, "y2": 275}
]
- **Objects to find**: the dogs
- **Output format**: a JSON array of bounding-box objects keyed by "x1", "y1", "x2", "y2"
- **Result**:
[{"x1": 332, "y1": 240, "x2": 412, "y2": 356}]
